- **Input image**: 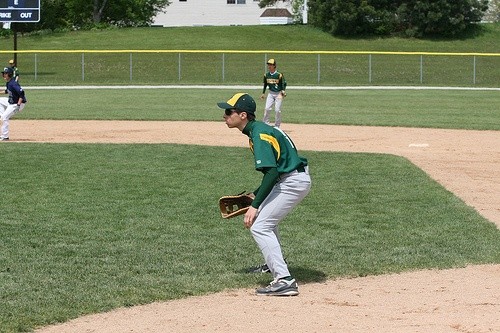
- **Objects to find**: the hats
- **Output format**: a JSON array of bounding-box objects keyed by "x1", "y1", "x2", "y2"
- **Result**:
[
  {"x1": 1, "y1": 67, "x2": 13, "y2": 75},
  {"x1": 217, "y1": 93, "x2": 257, "y2": 114},
  {"x1": 9, "y1": 59, "x2": 14, "y2": 64},
  {"x1": 267, "y1": 59, "x2": 276, "y2": 66}
]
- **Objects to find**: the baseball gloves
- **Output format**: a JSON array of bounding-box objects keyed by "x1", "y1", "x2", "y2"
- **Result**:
[{"x1": 218, "y1": 193, "x2": 255, "y2": 219}]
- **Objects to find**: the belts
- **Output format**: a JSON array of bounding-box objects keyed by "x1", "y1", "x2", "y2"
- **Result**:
[{"x1": 297, "y1": 167, "x2": 306, "y2": 173}]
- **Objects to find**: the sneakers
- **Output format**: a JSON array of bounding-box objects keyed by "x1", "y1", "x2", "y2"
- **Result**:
[
  {"x1": 247, "y1": 259, "x2": 287, "y2": 273},
  {"x1": 255, "y1": 278, "x2": 299, "y2": 295}
]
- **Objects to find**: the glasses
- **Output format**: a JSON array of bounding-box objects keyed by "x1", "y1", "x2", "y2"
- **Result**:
[{"x1": 225, "y1": 108, "x2": 241, "y2": 116}]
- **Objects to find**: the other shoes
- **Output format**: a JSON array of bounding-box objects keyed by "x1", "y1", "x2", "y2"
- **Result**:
[{"x1": 0, "y1": 136, "x2": 9, "y2": 141}]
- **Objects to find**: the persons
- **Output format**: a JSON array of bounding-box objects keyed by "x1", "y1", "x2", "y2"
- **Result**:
[
  {"x1": 217, "y1": 93, "x2": 312, "y2": 296},
  {"x1": 261, "y1": 59, "x2": 287, "y2": 128},
  {"x1": 0, "y1": 67, "x2": 27, "y2": 141},
  {"x1": 9, "y1": 59, "x2": 19, "y2": 82}
]
